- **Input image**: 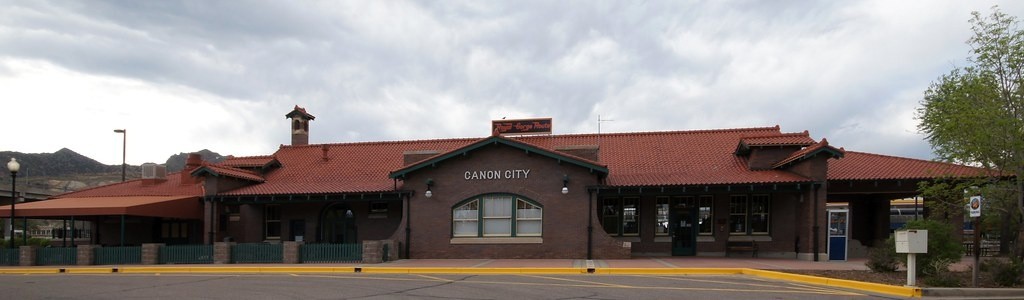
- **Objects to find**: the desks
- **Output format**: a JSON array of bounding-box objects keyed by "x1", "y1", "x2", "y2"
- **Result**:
[{"x1": 963, "y1": 243, "x2": 987, "y2": 257}]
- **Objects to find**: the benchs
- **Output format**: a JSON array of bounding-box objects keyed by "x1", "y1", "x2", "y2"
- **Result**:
[
  {"x1": 981, "y1": 247, "x2": 989, "y2": 249},
  {"x1": 726, "y1": 240, "x2": 759, "y2": 259}
]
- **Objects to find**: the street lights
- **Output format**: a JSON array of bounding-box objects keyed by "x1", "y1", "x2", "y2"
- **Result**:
[
  {"x1": 115, "y1": 129, "x2": 125, "y2": 181},
  {"x1": 7, "y1": 157, "x2": 21, "y2": 248}
]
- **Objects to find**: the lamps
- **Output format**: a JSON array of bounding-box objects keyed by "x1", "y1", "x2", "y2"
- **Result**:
[
  {"x1": 561, "y1": 175, "x2": 569, "y2": 194},
  {"x1": 425, "y1": 178, "x2": 433, "y2": 197}
]
